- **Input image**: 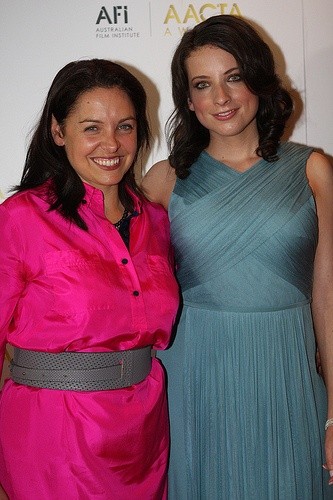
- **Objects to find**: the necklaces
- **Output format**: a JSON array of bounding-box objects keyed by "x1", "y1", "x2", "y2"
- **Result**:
[{"x1": 112, "y1": 203, "x2": 128, "y2": 227}]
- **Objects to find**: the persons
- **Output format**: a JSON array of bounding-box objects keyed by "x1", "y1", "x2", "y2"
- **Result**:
[
  {"x1": 140, "y1": 15, "x2": 333, "y2": 500},
  {"x1": 1, "y1": 58, "x2": 180, "y2": 500}
]
